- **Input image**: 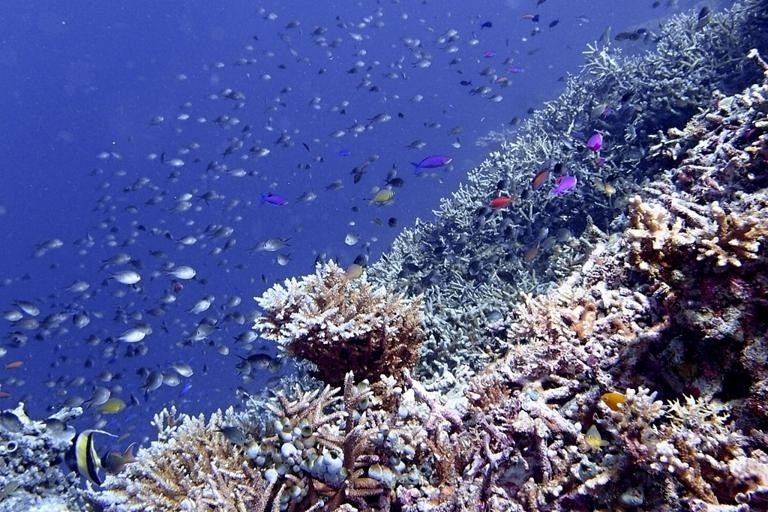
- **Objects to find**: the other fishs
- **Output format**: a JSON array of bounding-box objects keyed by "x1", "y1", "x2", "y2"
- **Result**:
[{"x1": 0, "y1": 0, "x2": 713, "y2": 511}]
